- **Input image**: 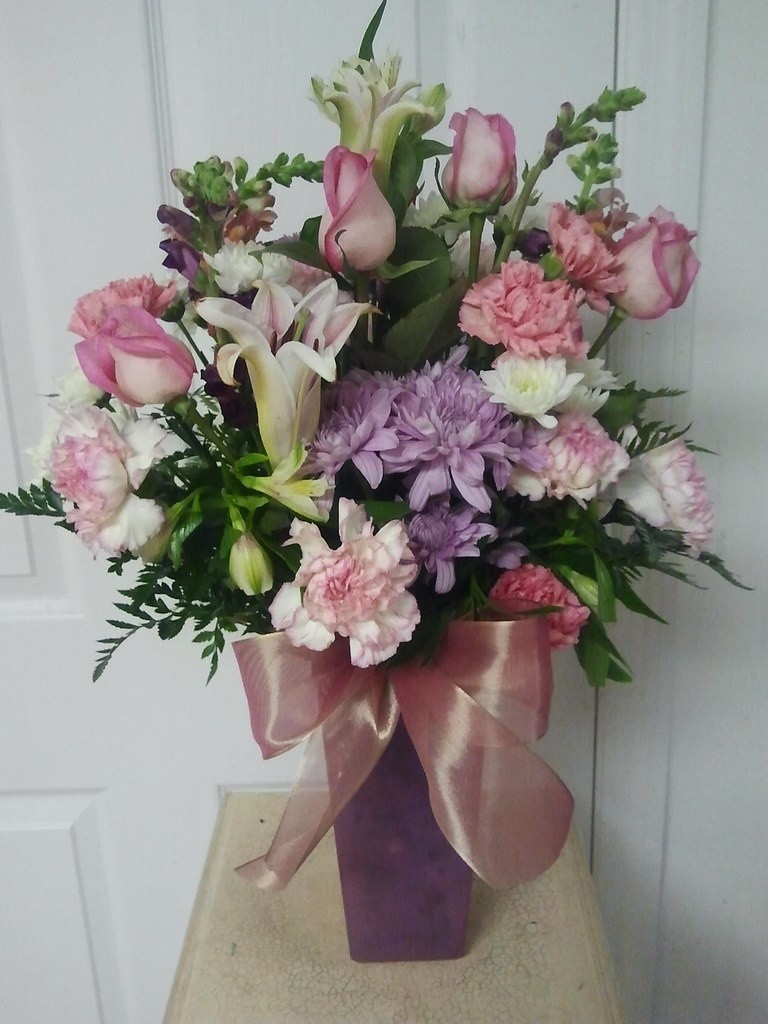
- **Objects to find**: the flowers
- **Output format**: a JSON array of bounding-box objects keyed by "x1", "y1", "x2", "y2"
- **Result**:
[{"x1": 0, "y1": 0, "x2": 768, "y2": 687}]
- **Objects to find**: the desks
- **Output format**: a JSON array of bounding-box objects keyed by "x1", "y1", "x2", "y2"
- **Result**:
[{"x1": 161, "y1": 792, "x2": 626, "y2": 1024}]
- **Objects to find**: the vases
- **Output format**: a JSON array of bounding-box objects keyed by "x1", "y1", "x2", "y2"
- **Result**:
[{"x1": 316, "y1": 664, "x2": 490, "y2": 964}]
